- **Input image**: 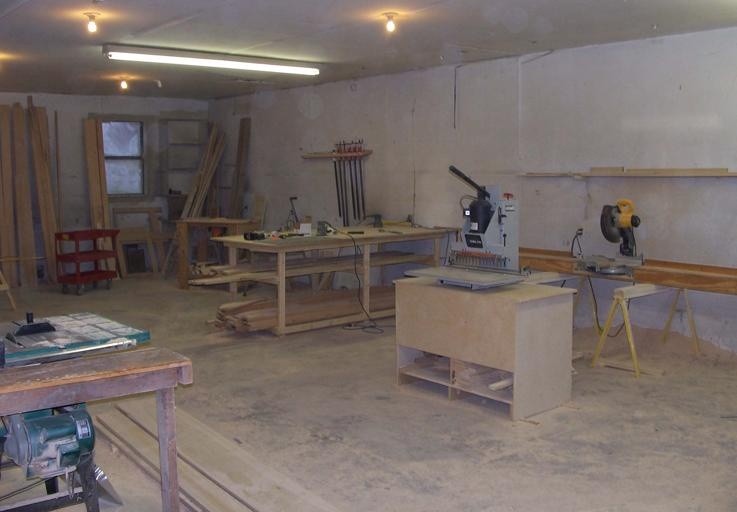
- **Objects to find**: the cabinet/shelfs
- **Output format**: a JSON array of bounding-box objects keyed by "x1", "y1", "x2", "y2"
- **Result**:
[
  {"x1": 391, "y1": 277, "x2": 577, "y2": 422},
  {"x1": 164, "y1": 116, "x2": 208, "y2": 198},
  {"x1": 209, "y1": 221, "x2": 461, "y2": 338},
  {"x1": 170, "y1": 216, "x2": 256, "y2": 293}
]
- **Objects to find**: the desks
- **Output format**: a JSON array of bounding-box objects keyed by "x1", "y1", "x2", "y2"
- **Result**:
[{"x1": 0, "y1": 341, "x2": 194, "y2": 512}]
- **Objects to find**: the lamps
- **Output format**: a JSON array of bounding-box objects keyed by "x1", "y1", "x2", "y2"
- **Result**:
[
  {"x1": 102, "y1": 43, "x2": 327, "y2": 79},
  {"x1": 84, "y1": 11, "x2": 100, "y2": 33},
  {"x1": 384, "y1": 12, "x2": 398, "y2": 32}
]
